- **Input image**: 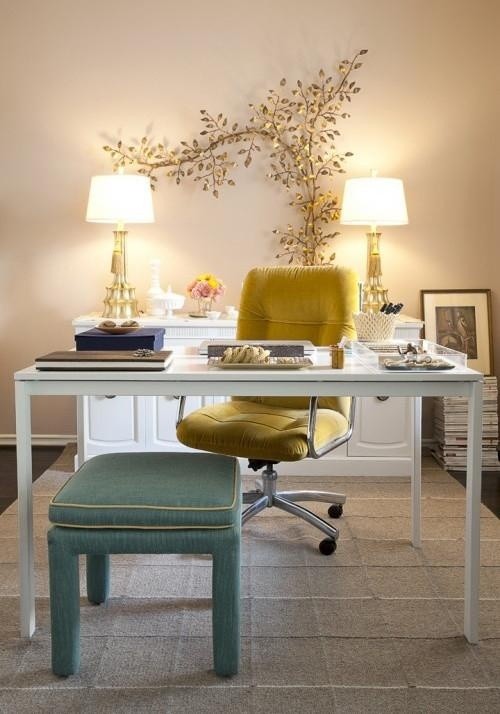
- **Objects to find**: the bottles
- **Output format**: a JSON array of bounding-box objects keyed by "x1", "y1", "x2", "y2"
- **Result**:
[{"x1": 145, "y1": 260, "x2": 164, "y2": 316}]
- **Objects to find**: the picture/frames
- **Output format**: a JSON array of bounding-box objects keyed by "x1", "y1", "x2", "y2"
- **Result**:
[{"x1": 419, "y1": 289, "x2": 494, "y2": 376}]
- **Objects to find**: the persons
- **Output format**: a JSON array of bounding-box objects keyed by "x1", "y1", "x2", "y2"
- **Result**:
[{"x1": 455, "y1": 309, "x2": 474, "y2": 353}]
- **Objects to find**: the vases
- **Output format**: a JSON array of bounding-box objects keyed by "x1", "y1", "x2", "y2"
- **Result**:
[{"x1": 198, "y1": 297, "x2": 212, "y2": 316}]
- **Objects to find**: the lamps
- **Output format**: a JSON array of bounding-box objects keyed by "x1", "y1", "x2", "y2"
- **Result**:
[
  {"x1": 84, "y1": 167, "x2": 155, "y2": 323},
  {"x1": 340, "y1": 168, "x2": 409, "y2": 314}
]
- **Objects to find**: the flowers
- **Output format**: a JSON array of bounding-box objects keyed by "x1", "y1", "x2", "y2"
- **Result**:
[{"x1": 187, "y1": 273, "x2": 226, "y2": 304}]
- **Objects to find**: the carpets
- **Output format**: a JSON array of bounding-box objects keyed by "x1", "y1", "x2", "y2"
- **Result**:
[{"x1": 0, "y1": 443, "x2": 499, "y2": 714}]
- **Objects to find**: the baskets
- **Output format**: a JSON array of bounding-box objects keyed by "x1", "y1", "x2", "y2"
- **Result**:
[{"x1": 352, "y1": 306, "x2": 400, "y2": 344}]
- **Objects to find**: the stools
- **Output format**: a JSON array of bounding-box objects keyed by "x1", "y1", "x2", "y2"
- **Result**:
[{"x1": 46, "y1": 453, "x2": 243, "y2": 679}]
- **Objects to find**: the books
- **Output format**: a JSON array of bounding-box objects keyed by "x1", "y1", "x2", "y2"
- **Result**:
[
  {"x1": 428, "y1": 373, "x2": 499, "y2": 473},
  {"x1": 34, "y1": 348, "x2": 174, "y2": 373},
  {"x1": 197, "y1": 338, "x2": 317, "y2": 358}
]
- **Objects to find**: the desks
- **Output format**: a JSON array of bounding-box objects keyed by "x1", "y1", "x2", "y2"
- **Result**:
[{"x1": 14, "y1": 345, "x2": 483, "y2": 644}]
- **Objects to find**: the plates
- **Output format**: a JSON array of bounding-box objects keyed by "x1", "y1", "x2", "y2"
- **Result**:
[
  {"x1": 95, "y1": 324, "x2": 144, "y2": 335},
  {"x1": 209, "y1": 356, "x2": 314, "y2": 371}
]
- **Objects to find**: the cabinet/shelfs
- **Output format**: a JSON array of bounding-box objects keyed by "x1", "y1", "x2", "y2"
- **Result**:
[{"x1": 73, "y1": 311, "x2": 425, "y2": 478}]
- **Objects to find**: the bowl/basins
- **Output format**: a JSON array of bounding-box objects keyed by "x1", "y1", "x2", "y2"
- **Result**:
[{"x1": 206, "y1": 312, "x2": 220, "y2": 322}]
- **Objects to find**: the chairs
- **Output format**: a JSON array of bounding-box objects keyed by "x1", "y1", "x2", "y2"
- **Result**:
[{"x1": 176, "y1": 267, "x2": 363, "y2": 555}]
